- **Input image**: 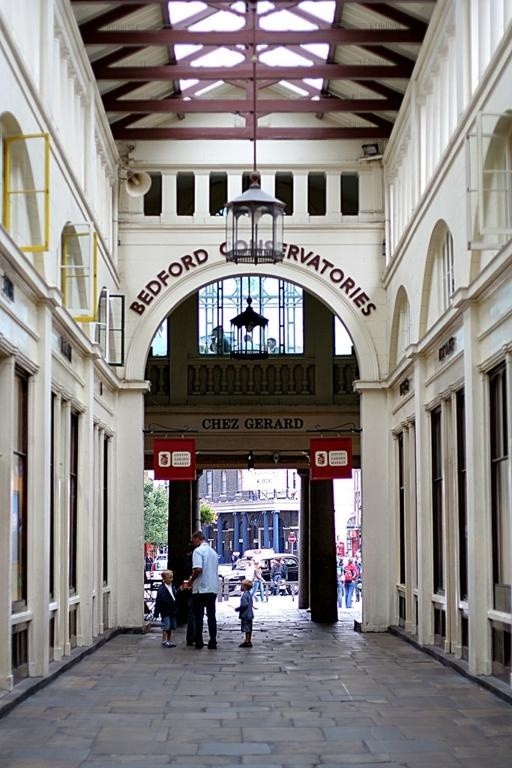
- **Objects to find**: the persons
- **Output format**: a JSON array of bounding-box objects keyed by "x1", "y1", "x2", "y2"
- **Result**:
[
  {"x1": 185, "y1": 531, "x2": 221, "y2": 650},
  {"x1": 243, "y1": 551, "x2": 361, "y2": 609},
  {"x1": 233, "y1": 579, "x2": 254, "y2": 648},
  {"x1": 152, "y1": 570, "x2": 185, "y2": 647},
  {"x1": 211, "y1": 325, "x2": 280, "y2": 354}
]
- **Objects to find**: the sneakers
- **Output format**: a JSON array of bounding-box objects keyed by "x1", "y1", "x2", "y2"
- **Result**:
[
  {"x1": 208, "y1": 640, "x2": 217, "y2": 649},
  {"x1": 161, "y1": 640, "x2": 177, "y2": 648},
  {"x1": 186, "y1": 640, "x2": 204, "y2": 649},
  {"x1": 239, "y1": 642, "x2": 252, "y2": 648}
]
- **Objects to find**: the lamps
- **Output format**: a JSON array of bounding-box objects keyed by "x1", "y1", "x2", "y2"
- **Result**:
[
  {"x1": 361, "y1": 142, "x2": 382, "y2": 164},
  {"x1": 247, "y1": 450, "x2": 256, "y2": 471},
  {"x1": 223, "y1": 1, "x2": 286, "y2": 361}
]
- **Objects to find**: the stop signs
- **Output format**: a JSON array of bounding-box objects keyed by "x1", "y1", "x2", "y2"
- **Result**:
[{"x1": 288, "y1": 535, "x2": 296, "y2": 543}]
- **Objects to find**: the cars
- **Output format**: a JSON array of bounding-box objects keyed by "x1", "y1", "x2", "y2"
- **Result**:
[
  {"x1": 144, "y1": 554, "x2": 168, "y2": 581},
  {"x1": 221, "y1": 549, "x2": 299, "y2": 597}
]
